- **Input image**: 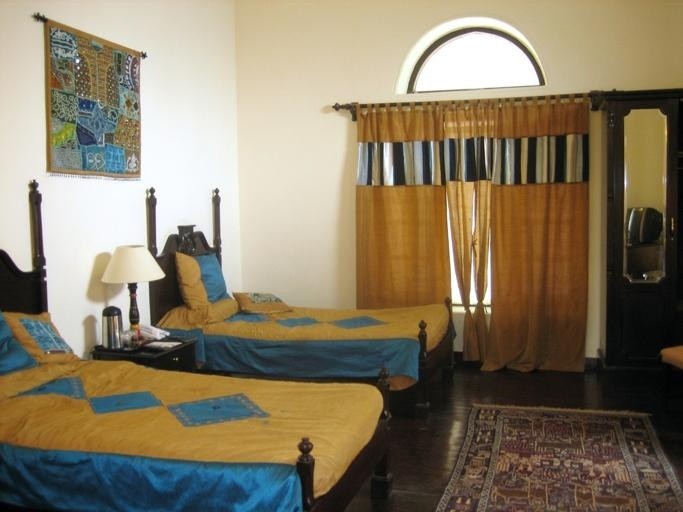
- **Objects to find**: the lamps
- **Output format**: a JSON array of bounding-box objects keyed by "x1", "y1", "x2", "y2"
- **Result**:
[{"x1": 100, "y1": 245, "x2": 166, "y2": 337}]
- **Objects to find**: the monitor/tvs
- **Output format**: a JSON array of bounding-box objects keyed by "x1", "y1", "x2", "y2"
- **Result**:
[{"x1": 625, "y1": 207, "x2": 663, "y2": 245}]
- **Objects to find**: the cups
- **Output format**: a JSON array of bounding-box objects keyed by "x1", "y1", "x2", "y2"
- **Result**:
[{"x1": 123, "y1": 330, "x2": 138, "y2": 351}]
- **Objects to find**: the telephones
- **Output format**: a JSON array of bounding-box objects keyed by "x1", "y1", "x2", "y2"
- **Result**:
[{"x1": 140, "y1": 323, "x2": 170, "y2": 341}]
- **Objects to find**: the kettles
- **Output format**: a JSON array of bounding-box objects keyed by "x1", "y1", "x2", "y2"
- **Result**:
[{"x1": 102, "y1": 306, "x2": 124, "y2": 351}]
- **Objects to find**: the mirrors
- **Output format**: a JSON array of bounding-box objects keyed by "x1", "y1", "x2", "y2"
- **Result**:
[{"x1": 622, "y1": 108, "x2": 666, "y2": 284}]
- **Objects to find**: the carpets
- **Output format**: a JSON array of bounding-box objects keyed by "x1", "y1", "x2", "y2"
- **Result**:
[{"x1": 434, "y1": 403, "x2": 683, "y2": 512}]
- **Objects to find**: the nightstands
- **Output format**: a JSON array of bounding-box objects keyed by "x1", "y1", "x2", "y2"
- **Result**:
[{"x1": 90, "y1": 333, "x2": 198, "y2": 372}]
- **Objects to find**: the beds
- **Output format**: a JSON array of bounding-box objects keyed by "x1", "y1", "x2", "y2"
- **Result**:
[
  {"x1": 146, "y1": 187, "x2": 457, "y2": 420},
  {"x1": 0, "y1": 179, "x2": 392, "y2": 512}
]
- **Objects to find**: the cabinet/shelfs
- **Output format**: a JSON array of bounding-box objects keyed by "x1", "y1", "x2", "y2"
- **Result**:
[{"x1": 598, "y1": 89, "x2": 683, "y2": 396}]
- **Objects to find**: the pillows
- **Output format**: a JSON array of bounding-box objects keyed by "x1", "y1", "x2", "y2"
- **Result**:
[
  {"x1": 174, "y1": 251, "x2": 226, "y2": 311},
  {"x1": 174, "y1": 295, "x2": 239, "y2": 322},
  {"x1": 3, "y1": 310, "x2": 80, "y2": 364},
  {"x1": 233, "y1": 292, "x2": 292, "y2": 313}
]
